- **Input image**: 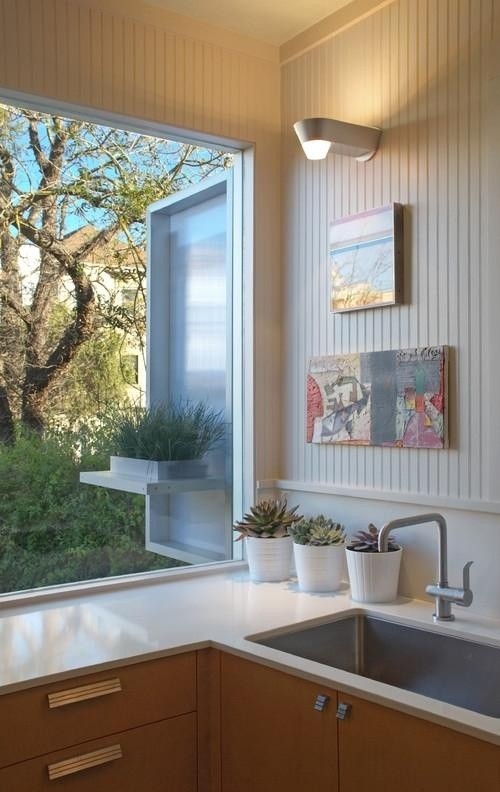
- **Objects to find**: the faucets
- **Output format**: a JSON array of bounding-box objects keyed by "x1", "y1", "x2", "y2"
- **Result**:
[{"x1": 377, "y1": 512, "x2": 473, "y2": 622}]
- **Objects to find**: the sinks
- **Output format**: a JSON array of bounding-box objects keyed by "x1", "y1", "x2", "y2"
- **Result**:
[{"x1": 242, "y1": 607, "x2": 500, "y2": 720}]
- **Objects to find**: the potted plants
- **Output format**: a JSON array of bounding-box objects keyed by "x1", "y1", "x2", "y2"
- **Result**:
[
  {"x1": 346, "y1": 524, "x2": 403, "y2": 601},
  {"x1": 233, "y1": 497, "x2": 302, "y2": 581},
  {"x1": 287, "y1": 515, "x2": 344, "y2": 592}
]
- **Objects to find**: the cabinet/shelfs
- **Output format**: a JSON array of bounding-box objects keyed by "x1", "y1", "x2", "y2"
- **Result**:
[{"x1": 0, "y1": 649, "x2": 500, "y2": 792}]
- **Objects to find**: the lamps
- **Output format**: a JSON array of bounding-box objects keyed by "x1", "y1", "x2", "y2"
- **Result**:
[{"x1": 294, "y1": 117, "x2": 382, "y2": 162}]
